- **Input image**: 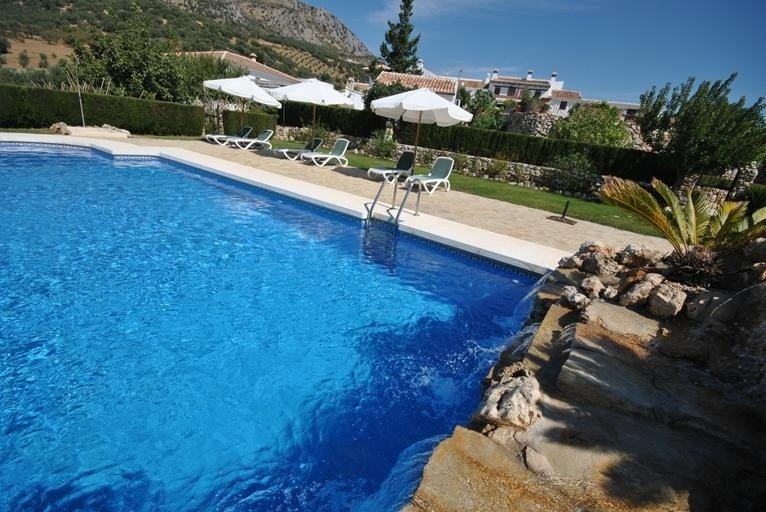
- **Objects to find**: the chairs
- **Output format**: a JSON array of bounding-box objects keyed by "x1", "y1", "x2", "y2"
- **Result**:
[{"x1": 203, "y1": 126, "x2": 455, "y2": 197}]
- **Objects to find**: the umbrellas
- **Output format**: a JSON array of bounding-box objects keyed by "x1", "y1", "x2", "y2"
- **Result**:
[
  {"x1": 202, "y1": 74, "x2": 283, "y2": 138},
  {"x1": 267, "y1": 79, "x2": 356, "y2": 151},
  {"x1": 369, "y1": 85, "x2": 475, "y2": 175}
]
